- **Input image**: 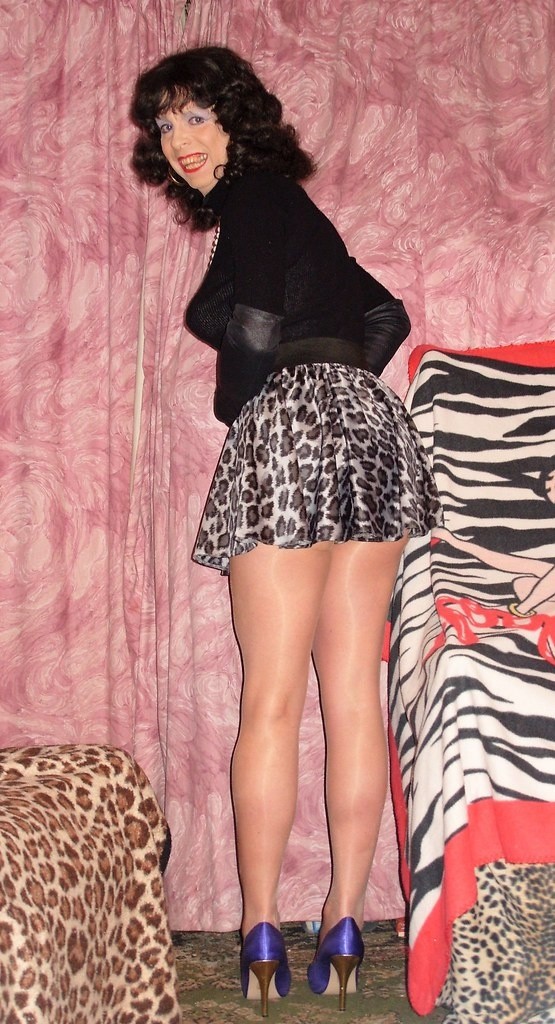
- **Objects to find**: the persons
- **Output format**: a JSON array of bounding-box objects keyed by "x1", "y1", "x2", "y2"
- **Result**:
[{"x1": 129, "y1": 47, "x2": 445, "y2": 1019}]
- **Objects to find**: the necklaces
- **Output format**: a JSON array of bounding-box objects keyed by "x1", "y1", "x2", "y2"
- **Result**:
[{"x1": 207, "y1": 219, "x2": 221, "y2": 265}]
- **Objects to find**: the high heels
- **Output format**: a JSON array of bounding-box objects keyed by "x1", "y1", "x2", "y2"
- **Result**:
[
  {"x1": 240, "y1": 921, "x2": 291, "y2": 1017},
  {"x1": 307, "y1": 916, "x2": 365, "y2": 1011}
]
